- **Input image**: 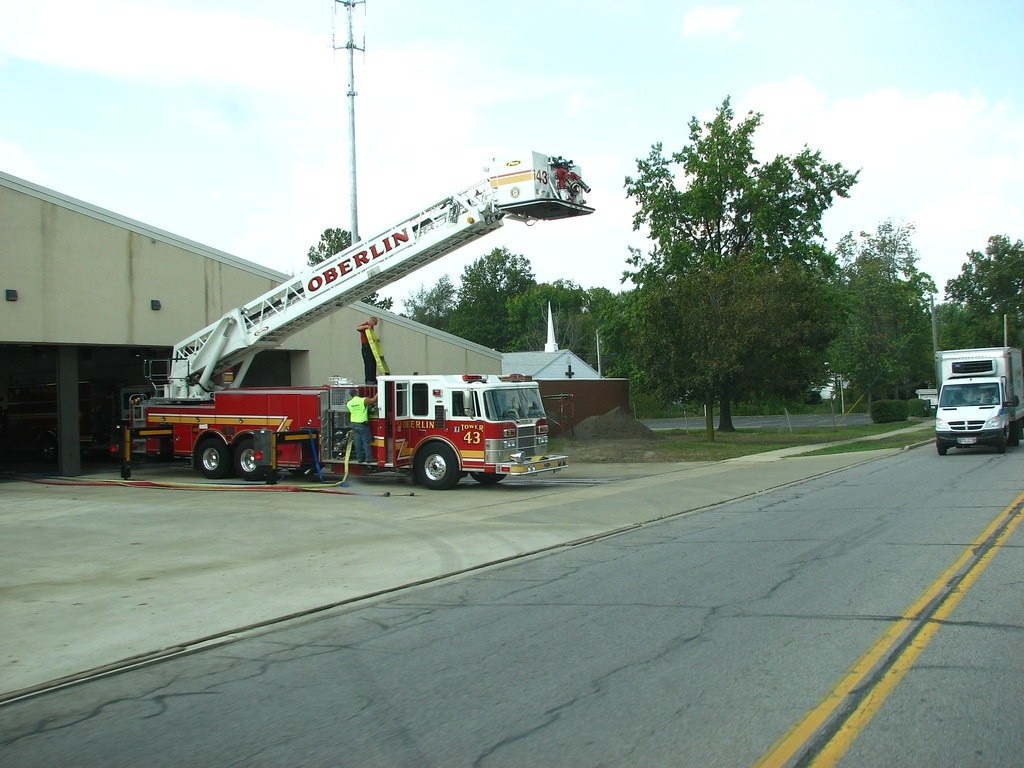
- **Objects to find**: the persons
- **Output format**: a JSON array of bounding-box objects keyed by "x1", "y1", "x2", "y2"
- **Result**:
[
  {"x1": 347, "y1": 389, "x2": 377, "y2": 463},
  {"x1": 357, "y1": 317, "x2": 379, "y2": 385},
  {"x1": 979, "y1": 388, "x2": 999, "y2": 404}
]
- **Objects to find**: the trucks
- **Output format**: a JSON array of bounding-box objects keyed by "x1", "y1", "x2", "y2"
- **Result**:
[{"x1": 925, "y1": 346, "x2": 1024, "y2": 456}]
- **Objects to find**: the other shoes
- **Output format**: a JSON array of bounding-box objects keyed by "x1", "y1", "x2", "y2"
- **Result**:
[
  {"x1": 365, "y1": 380, "x2": 375, "y2": 385},
  {"x1": 367, "y1": 459, "x2": 378, "y2": 463},
  {"x1": 358, "y1": 459, "x2": 365, "y2": 463}
]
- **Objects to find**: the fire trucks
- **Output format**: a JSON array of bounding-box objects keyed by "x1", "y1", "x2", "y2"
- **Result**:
[
  {"x1": 110, "y1": 151, "x2": 596, "y2": 491},
  {"x1": 0, "y1": 377, "x2": 164, "y2": 468}
]
